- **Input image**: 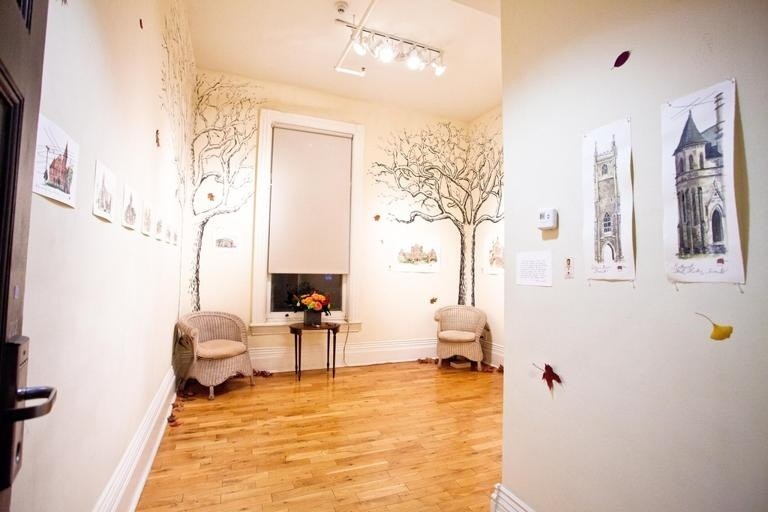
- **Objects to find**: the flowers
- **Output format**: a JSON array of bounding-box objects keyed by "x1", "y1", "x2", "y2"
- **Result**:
[{"x1": 296, "y1": 291, "x2": 332, "y2": 316}]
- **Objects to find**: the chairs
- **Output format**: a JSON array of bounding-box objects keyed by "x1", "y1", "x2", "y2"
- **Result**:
[
  {"x1": 174, "y1": 311, "x2": 256, "y2": 400},
  {"x1": 434, "y1": 305, "x2": 488, "y2": 372}
]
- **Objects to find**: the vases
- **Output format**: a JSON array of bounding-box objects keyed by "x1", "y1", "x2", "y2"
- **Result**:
[{"x1": 304, "y1": 310, "x2": 321, "y2": 325}]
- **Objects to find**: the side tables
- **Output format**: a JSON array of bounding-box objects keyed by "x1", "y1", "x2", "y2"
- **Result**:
[{"x1": 288, "y1": 321, "x2": 341, "y2": 382}]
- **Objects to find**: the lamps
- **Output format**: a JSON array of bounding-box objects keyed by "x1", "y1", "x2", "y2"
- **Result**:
[{"x1": 335, "y1": 17, "x2": 447, "y2": 79}]
